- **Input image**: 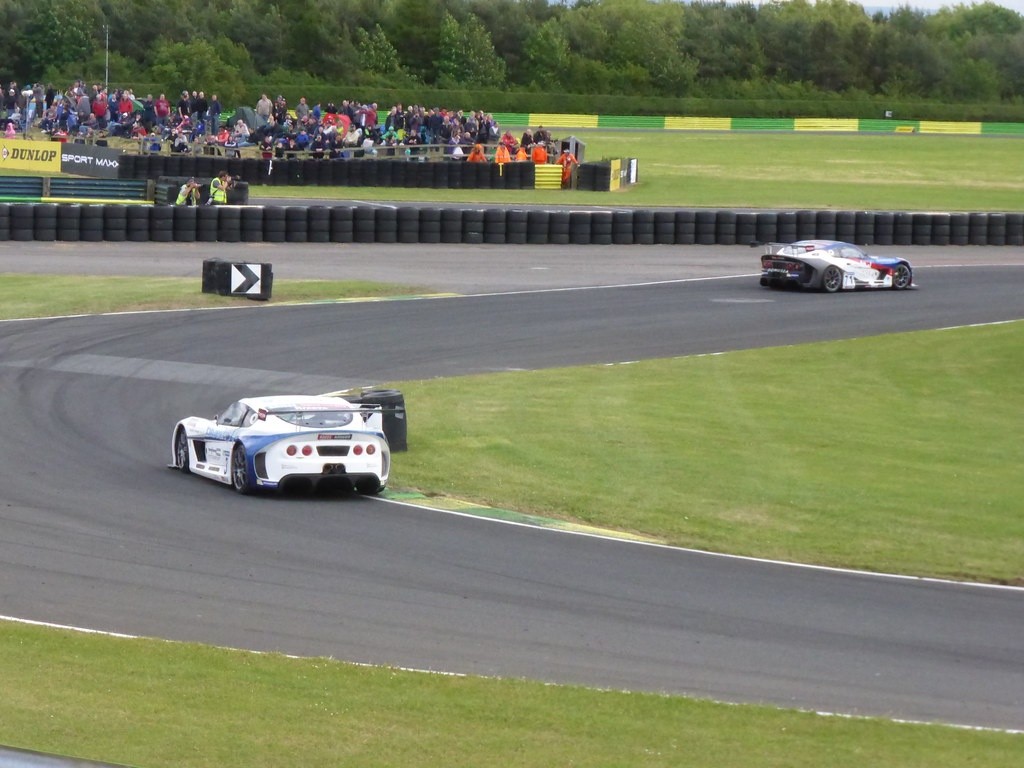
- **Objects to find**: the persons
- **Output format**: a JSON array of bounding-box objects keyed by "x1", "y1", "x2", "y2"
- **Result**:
[
  {"x1": 174, "y1": 176, "x2": 200, "y2": 206},
  {"x1": 554, "y1": 149, "x2": 581, "y2": 188},
  {"x1": 210, "y1": 170, "x2": 232, "y2": 205},
  {"x1": 0, "y1": 75, "x2": 558, "y2": 170}
]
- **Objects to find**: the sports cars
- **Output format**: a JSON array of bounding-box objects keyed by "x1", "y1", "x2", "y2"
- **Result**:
[
  {"x1": 168, "y1": 395, "x2": 392, "y2": 498},
  {"x1": 760, "y1": 239, "x2": 913, "y2": 293}
]
